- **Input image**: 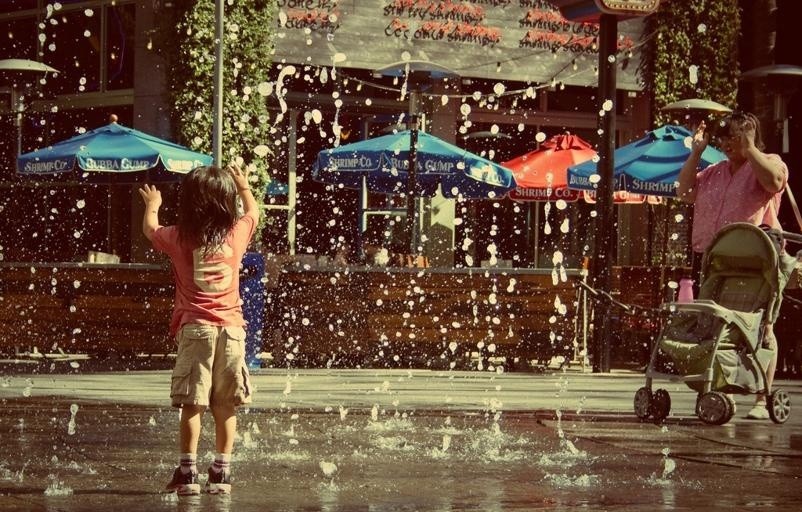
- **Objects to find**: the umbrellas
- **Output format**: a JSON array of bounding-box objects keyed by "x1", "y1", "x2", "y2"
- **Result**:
[
  {"x1": 15, "y1": 111, "x2": 291, "y2": 256},
  {"x1": 311, "y1": 126, "x2": 517, "y2": 201},
  {"x1": 498, "y1": 130, "x2": 663, "y2": 205},
  {"x1": 567, "y1": 123, "x2": 731, "y2": 197}
]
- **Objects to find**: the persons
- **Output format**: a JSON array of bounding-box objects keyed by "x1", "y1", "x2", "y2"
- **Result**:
[
  {"x1": 677, "y1": 110, "x2": 790, "y2": 419},
  {"x1": 137, "y1": 162, "x2": 260, "y2": 494}
]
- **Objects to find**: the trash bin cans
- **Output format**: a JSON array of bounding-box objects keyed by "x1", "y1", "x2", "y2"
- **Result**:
[{"x1": 238, "y1": 252, "x2": 265, "y2": 368}]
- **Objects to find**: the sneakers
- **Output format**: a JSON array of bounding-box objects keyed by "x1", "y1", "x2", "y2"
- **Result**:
[
  {"x1": 164, "y1": 468, "x2": 201, "y2": 496},
  {"x1": 204, "y1": 467, "x2": 233, "y2": 495},
  {"x1": 747, "y1": 400, "x2": 770, "y2": 421}
]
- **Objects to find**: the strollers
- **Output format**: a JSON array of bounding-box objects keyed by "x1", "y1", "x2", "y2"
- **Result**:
[{"x1": 632, "y1": 221, "x2": 802, "y2": 426}]
